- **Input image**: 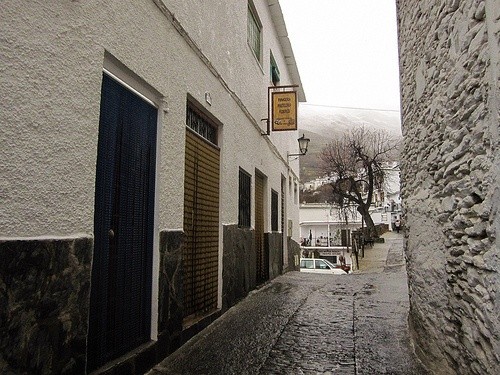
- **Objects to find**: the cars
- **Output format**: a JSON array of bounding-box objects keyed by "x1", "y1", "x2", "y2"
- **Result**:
[{"x1": 300, "y1": 258, "x2": 347, "y2": 275}]
